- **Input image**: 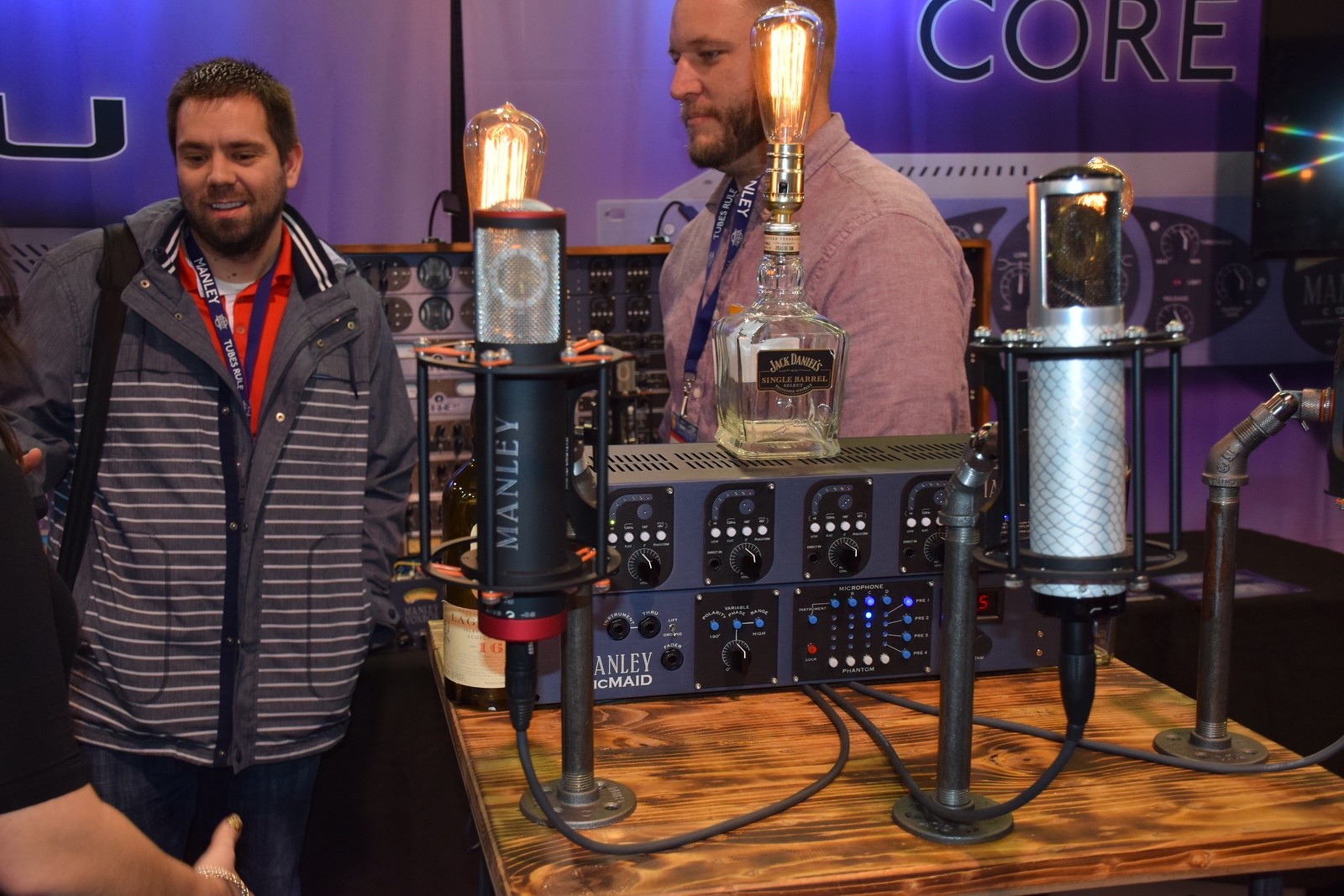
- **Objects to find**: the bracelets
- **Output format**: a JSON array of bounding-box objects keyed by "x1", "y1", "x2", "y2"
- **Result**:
[{"x1": 194, "y1": 865, "x2": 249, "y2": 896}]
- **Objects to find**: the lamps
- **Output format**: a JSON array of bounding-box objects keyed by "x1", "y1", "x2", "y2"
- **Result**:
[
  {"x1": 751, "y1": 1, "x2": 823, "y2": 223},
  {"x1": 466, "y1": 100, "x2": 549, "y2": 240},
  {"x1": 1061, "y1": 156, "x2": 1133, "y2": 264}
]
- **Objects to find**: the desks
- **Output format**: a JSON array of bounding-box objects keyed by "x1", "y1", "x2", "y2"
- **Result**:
[{"x1": 425, "y1": 617, "x2": 1343, "y2": 896}]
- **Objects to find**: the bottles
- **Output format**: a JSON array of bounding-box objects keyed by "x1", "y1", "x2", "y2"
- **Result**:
[
  {"x1": 438, "y1": 378, "x2": 514, "y2": 712},
  {"x1": 711, "y1": 223, "x2": 850, "y2": 460}
]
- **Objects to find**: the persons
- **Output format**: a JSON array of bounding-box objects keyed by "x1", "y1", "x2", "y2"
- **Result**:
[
  {"x1": 0, "y1": 425, "x2": 241, "y2": 896},
  {"x1": 15, "y1": 55, "x2": 423, "y2": 896},
  {"x1": 657, "y1": 0, "x2": 972, "y2": 440}
]
